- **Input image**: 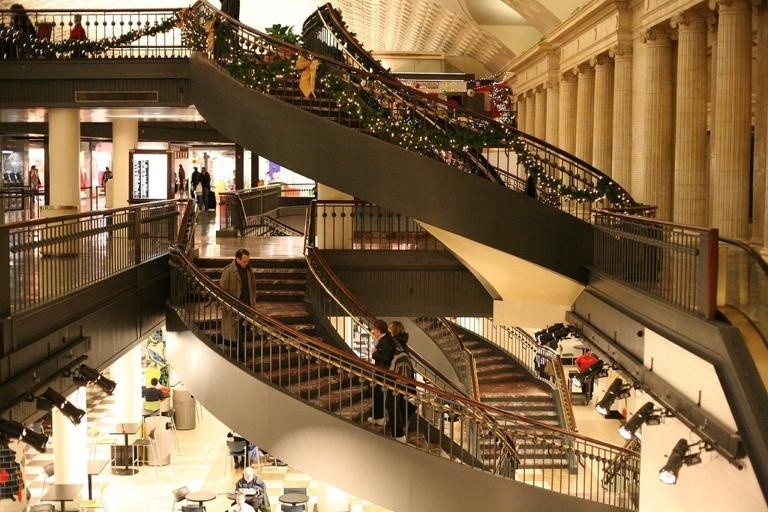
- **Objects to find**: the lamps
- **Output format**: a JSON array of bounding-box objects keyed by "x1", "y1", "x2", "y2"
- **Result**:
[
  {"x1": 0, "y1": 358, "x2": 117, "y2": 452},
  {"x1": 533, "y1": 319, "x2": 714, "y2": 487}
]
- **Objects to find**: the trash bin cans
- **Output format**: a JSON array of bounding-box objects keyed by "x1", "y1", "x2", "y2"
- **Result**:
[
  {"x1": 173, "y1": 389, "x2": 197, "y2": 429},
  {"x1": 145, "y1": 416, "x2": 172, "y2": 466},
  {"x1": 37, "y1": 205, "x2": 78, "y2": 259}
]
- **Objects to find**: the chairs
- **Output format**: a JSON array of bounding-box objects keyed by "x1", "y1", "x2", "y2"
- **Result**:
[
  {"x1": 130, "y1": 424, "x2": 163, "y2": 476},
  {"x1": 173, "y1": 431, "x2": 310, "y2": 512},
  {"x1": 25, "y1": 488, "x2": 54, "y2": 512}
]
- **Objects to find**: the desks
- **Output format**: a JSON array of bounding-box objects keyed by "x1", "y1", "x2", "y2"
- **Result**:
[{"x1": 39, "y1": 423, "x2": 142, "y2": 512}]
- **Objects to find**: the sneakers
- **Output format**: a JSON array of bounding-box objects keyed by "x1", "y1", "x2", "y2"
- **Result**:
[
  {"x1": 367, "y1": 416, "x2": 386, "y2": 426},
  {"x1": 395, "y1": 434, "x2": 406, "y2": 443}
]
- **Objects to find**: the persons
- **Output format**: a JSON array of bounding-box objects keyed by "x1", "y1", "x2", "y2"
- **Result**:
[
  {"x1": 30, "y1": 165, "x2": 41, "y2": 202},
  {"x1": 365, "y1": 319, "x2": 409, "y2": 444},
  {"x1": 228, "y1": 493, "x2": 255, "y2": 512},
  {"x1": 197, "y1": 167, "x2": 211, "y2": 214},
  {"x1": 234, "y1": 467, "x2": 272, "y2": 512},
  {"x1": 535, "y1": 339, "x2": 552, "y2": 378},
  {"x1": 387, "y1": 321, "x2": 420, "y2": 425},
  {"x1": 141, "y1": 379, "x2": 169, "y2": 417},
  {"x1": 574, "y1": 348, "x2": 599, "y2": 395},
  {"x1": 67, "y1": 14, "x2": 89, "y2": 56},
  {"x1": 101, "y1": 167, "x2": 113, "y2": 194},
  {"x1": 226, "y1": 430, "x2": 251, "y2": 469},
  {"x1": 5, "y1": 4, "x2": 38, "y2": 59},
  {"x1": 179, "y1": 164, "x2": 186, "y2": 191},
  {"x1": 218, "y1": 248, "x2": 258, "y2": 363},
  {"x1": 190, "y1": 166, "x2": 201, "y2": 190}
]
